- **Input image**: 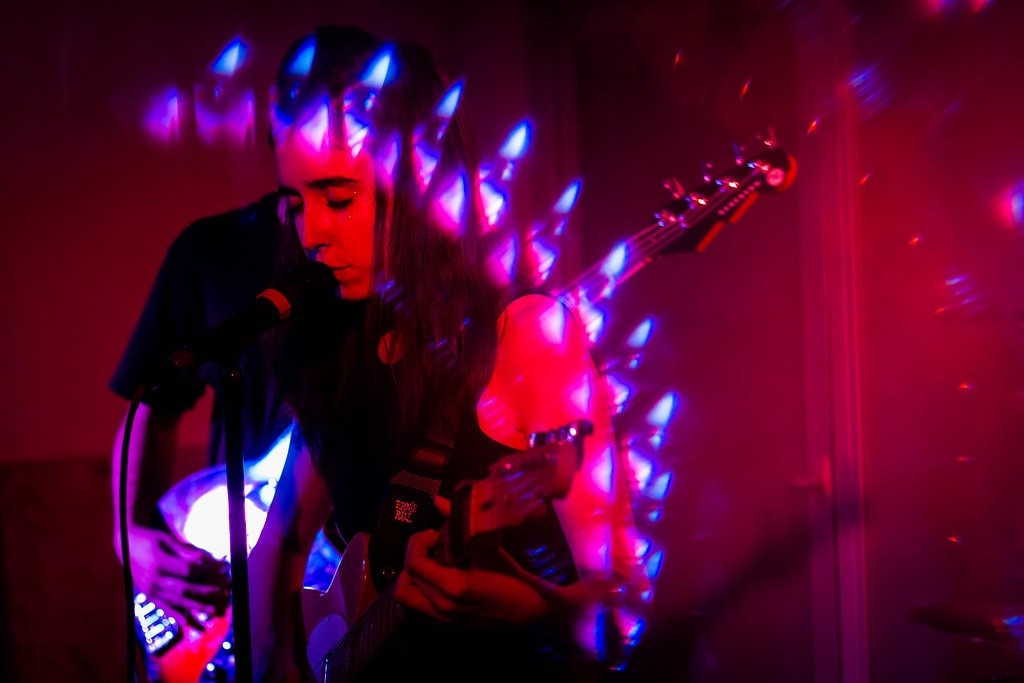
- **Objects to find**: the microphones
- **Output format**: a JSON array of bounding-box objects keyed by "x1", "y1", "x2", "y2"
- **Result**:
[{"x1": 188, "y1": 263, "x2": 333, "y2": 363}]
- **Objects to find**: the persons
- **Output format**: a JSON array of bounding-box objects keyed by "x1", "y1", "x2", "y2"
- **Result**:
[
  {"x1": 107, "y1": 26, "x2": 373, "y2": 627},
  {"x1": 269, "y1": 41, "x2": 655, "y2": 683}
]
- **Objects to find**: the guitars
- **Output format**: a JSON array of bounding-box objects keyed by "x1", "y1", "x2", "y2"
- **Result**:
[
  {"x1": 291, "y1": 415, "x2": 593, "y2": 683},
  {"x1": 130, "y1": 122, "x2": 796, "y2": 683}
]
